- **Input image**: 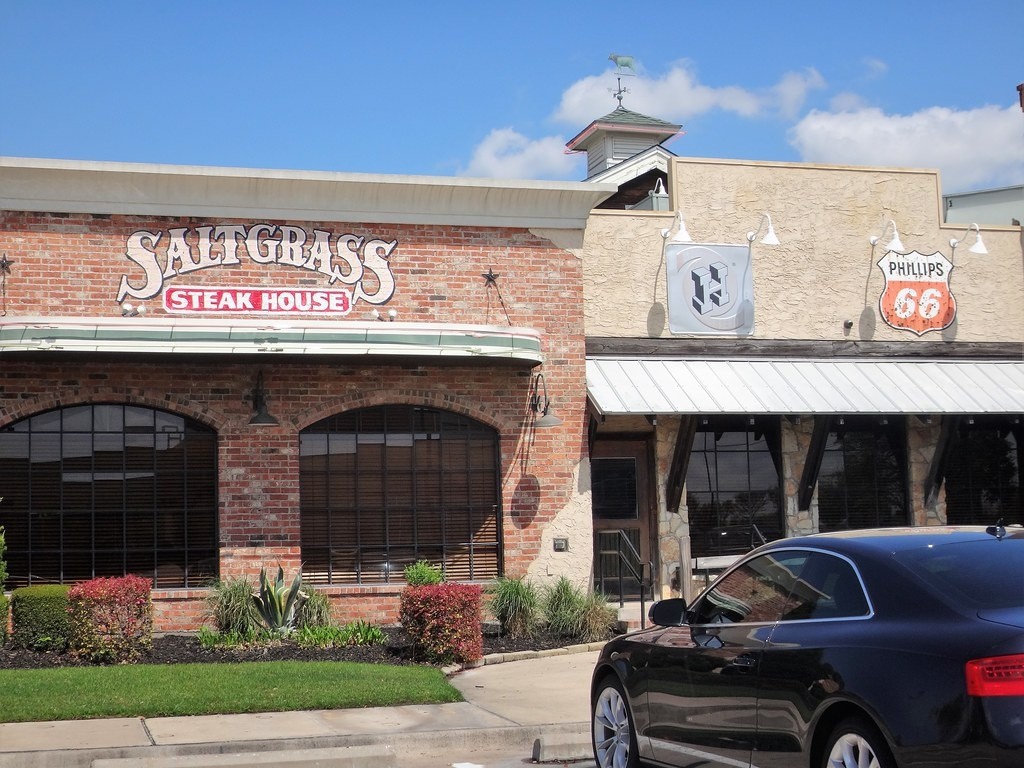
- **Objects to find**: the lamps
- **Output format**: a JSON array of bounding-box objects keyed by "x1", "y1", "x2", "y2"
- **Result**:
[
  {"x1": 869, "y1": 220, "x2": 906, "y2": 251},
  {"x1": 745, "y1": 212, "x2": 779, "y2": 246},
  {"x1": 950, "y1": 221, "x2": 990, "y2": 254},
  {"x1": 249, "y1": 369, "x2": 279, "y2": 429},
  {"x1": 530, "y1": 372, "x2": 565, "y2": 429},
  {"x1": 658, "y1": 211, "x2": 692, "y2": 243}
]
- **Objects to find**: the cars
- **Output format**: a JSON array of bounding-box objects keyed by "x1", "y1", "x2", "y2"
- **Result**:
[{"x1": 591, "y1": 524, "x2": 1024, "y2": 768}]
